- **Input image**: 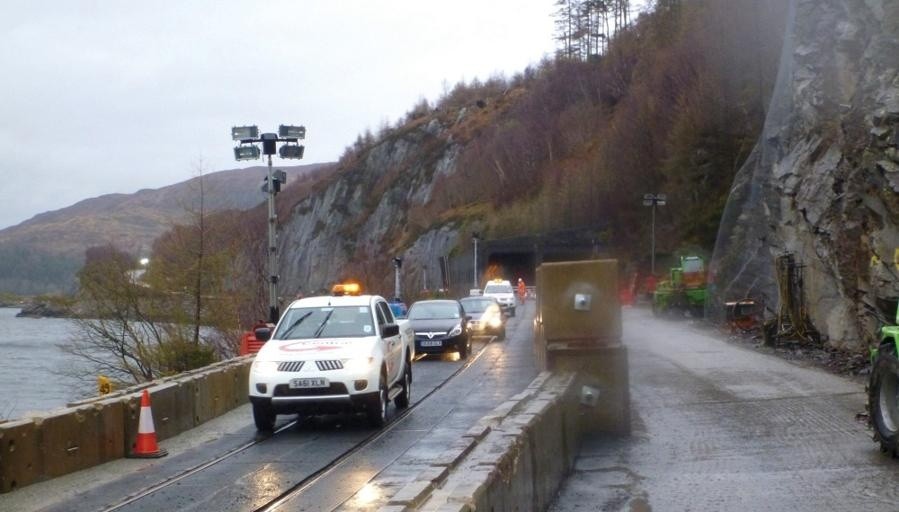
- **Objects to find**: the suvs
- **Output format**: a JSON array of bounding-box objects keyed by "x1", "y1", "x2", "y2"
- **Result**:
[{"x1": 483, "y1": 279, "x2": 516, "y2": 316}]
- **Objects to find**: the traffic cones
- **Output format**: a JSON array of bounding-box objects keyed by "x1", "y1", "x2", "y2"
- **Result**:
[{"x1": 129, "y1": 390, "x2": 167, "y2": 458}]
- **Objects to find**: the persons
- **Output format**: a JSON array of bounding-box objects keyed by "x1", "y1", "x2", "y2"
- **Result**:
[
  {"x1": 643, "y1": 271, "x2": 659, "y2": 302},
  {"x1": 517, "y1": 278, "x2": 526, "y2": 306}
]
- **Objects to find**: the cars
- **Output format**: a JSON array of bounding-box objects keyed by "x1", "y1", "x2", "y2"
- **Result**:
[
  {"x1": 460, "y1": 297, "x2": 505, "y2": 339},
  {"x1": 406, "y1": 300, "x2": 472, "y2": 358}
]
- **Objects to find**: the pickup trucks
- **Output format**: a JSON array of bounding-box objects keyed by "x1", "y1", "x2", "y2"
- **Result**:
[{"x1": 247, "y1": 295, "x2": 414, "y2": 430}]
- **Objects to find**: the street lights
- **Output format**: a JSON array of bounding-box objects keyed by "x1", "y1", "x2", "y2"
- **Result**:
[
  {"x1": 472, "y1": 230, "x2": 481, "y2": 288},
  {"x1": 642, "y1": 191, "x2": 668, "y2": 275},
  {"x1": 231, "y1": 124, "x2": 305, "y2": 327}
]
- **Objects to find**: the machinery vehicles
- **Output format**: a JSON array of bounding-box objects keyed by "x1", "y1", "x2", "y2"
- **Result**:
[{"x1": 652, "y1": 255, "x2": 709, "y2": 319}]
